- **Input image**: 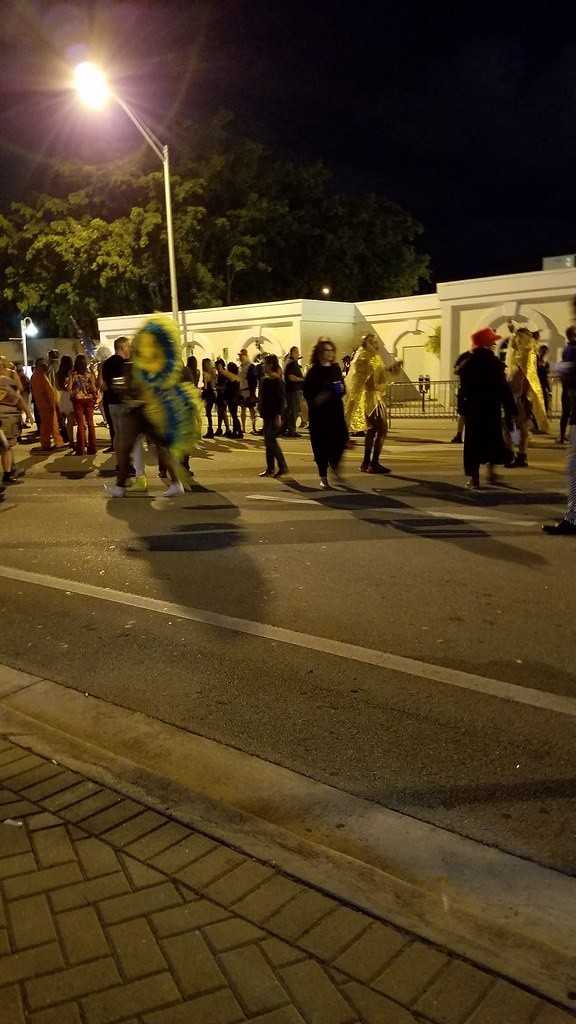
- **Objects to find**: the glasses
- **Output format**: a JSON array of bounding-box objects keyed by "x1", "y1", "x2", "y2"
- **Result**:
[{"x1": 322, "y1": 349, "x2": 335, "y2": 351}]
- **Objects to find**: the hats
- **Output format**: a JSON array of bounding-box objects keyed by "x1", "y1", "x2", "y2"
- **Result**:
[
  {"x1": 237, "y1": 348, "x2": 248, "y2": 355},
  {"x1": 469, "y1": 327, "x2": 502, "y2": 351}
]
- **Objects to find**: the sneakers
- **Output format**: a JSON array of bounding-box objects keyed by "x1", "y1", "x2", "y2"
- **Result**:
[
  {"x1": 103, "y1": 485, "x2": 126, "y2": 498},
  {"x1": 162, "y1": 480, "x2": 185, "y2": 497},
  {"x1": 464, "y1": 480, "x2": 480, "y2": 492},
  {"x1": 126, "y1": 475, "x2": 148, "y2": 492}
]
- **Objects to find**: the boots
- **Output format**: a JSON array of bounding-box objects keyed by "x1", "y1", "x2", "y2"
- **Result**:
[
  {"x1": 202, "y1": 427, "x2": 214, "y2": 439},
  {"x1": 223, "y1": 430, "x2": 231, "y2": 437},
  {"x1": 213, "y1": 428, "x2": 222, "y2": 436},
  {"x1": 503, "y1": 451, "x2": 528, "y2": 468}
]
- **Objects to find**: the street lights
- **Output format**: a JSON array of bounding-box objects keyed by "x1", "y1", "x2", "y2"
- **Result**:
[
  {"x1": 75, "y1": 63, "x2": 178, "y2": 329},
  {"x1": 20, "y1": 316, "x2": 38, "y2": 374}
]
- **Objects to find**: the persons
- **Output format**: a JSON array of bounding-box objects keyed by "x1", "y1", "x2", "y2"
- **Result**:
[
  {"x1": 302, "y1": 336, "x2": 348, "y2": 489},
  {"x1": 185, "y1": 356, "x2": 200, "y2": 388},
  {"x1": 541, "y1": 397, "x2": 576, "y2": 537},
  {"x1": 101, "y1": 337, "x2": 136, "y2": 487},
  {"x1": 214, "y1": 359, "x2": 232, "y2": 437},
  {"x1": 341, "y1": 330, "x2": 402, "y2": 474},
  {"x1": 0, "y1": 346, "x2": 112, "y2": 501},
  {"x1": 449, "y1": 320, "x2": 553, "y2": 467},
  {"x1": 454, "y1": 328, "x2": 514, "y2": 488},
  {"x1": 280, "y1": 345, "x2": 304, "y2": 438},
  {"x1": 55, "y1": 354, "x2": 78, "y2": 449},
  {"x1": 237, "y1": 349, "x2": 258, "y2": 435},
  {"x1": 224, "y1": 360, "x2": 244, "y2": 439},
  {"x1": 555, "y1": 325, "x2": 576, "y2": 445},
  {"x1": 256, "y1": 352, "x2": 289, "y2": 479},
  {"x1": 157, "y1": 446, "x2": 194, "y2": 477},
  {"x1": 65, "y1": 353, "x2": 97, "y2": 455},
  {"x1": 200, "y1": 358, "x2": 216, "y2": 439},
  {"x1": 30, "y1": 358, "x2": 64, "y2": 451}
]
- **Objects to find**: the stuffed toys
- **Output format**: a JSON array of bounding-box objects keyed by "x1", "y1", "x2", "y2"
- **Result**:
[{"x1": 122, "y1": 311, "x2": 205, "y2": 495}]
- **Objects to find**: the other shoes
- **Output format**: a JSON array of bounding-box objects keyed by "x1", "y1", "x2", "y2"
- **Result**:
[
  {"x1": 451, "y1": 432, "x2": 463, "y2": 443},
  {"x1": 287, "y1": 432, "x2": 302, "y2": 437},
  {"x1": 319, "y1": 477, "x2": 329, "y2": 488},
  {"x1": 366, "y1": 463, "x2": 392, "y2": 474},
  {"x1": 68, "y1": 443, "x2": 77, "y2": 451},
  {"x1": 270, "y1": 468, "x2": 289, "y2": 479},
  {"x1": 555, "y1": 438, "x2": 564, "y2": 444},
  {"x1": 540, "y1": 520, "x2": 575, "y2": 535},
  {"x1": 358, "y1": 461, "x2": 371, "y2": 472},
  {"x1": 241, "y1": 430, "x2": 246, "y2": 433},
  {"x1": 259, "y1": 470, "x2": 275, "y2": 477},
  {"x1": 249, "y1": 429, "x2": 256, "y2": 434},
  {"x1": 103, "y1": 446, "x2": 115, "y2": 454},
  {"x1": 0, "y1": 462, "x2": 25, "y2": 501},
  {"x1": 280, "y1": 430, "x2": 288, "y2": 437},
  {"x1": 230, "y1": 433, "x2": 244, "y2": 439}
]
